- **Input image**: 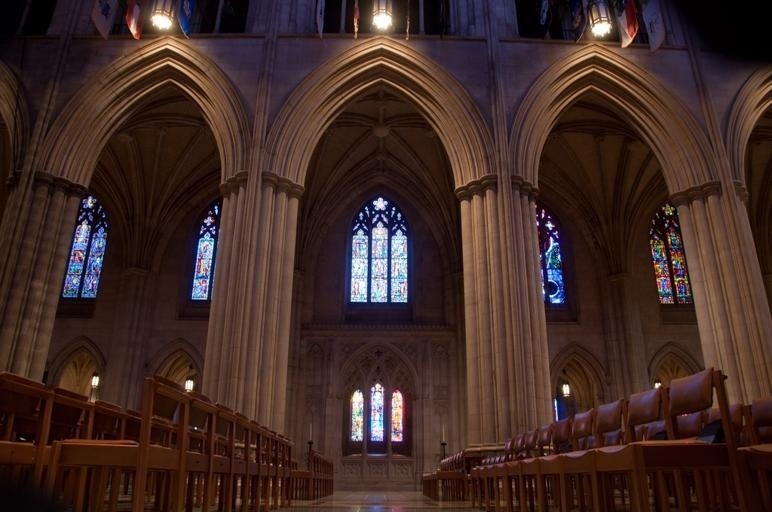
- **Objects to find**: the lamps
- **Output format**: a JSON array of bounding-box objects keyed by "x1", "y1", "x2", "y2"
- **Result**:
[
  {"x1": 146, "y1": 0, "x2": 177, "y2": 31},
  {"x1": 369, "y1": 0, "x2": 396, "y2": 32},
  {"x1": 586, "y1": 1, "x2": 613, "y2": 39}
]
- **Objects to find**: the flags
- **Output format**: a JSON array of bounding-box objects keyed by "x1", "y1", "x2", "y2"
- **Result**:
[
  {"x1": 567, "y1": 1, "x2": 587, "y2": 44},
  {"x1": 538, "y1": 1, "x2": 554, "y2": 34},
  {"x1": 612, "y1": 0, "x2": 639, "y2": 49},
  {"x1": 642, "y1": 0, "x2": 666, "y2": 52},
  {"x1": 176, "y1": 0, "x2": 197, "y2": 39},
  {"x1": 124, "y1": 0, "x2": 153, "y2": 39},
  {"x1": 315, "y1": 0, "x2": 326, "y2": 40},
  {"x1": 403, "y1": 1, "x2": 412, "y2": 40},
  {"x1": 439, "y1": 0, "x2": 446, "y2": 40},
  {"x1": 353, "y1": 1, "x2": 361, "y2": 39},
  {"x1": 90, "y1": 0, "x2": 119, "y2": 39}
]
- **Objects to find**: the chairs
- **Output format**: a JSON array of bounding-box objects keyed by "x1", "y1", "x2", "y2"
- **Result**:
[
  {"x1": 0, "y1": 370, "x2": 334, "y2": 512},
  {"x1": 421, "y1": 364, "x2": 772, "y2": 512}
]
